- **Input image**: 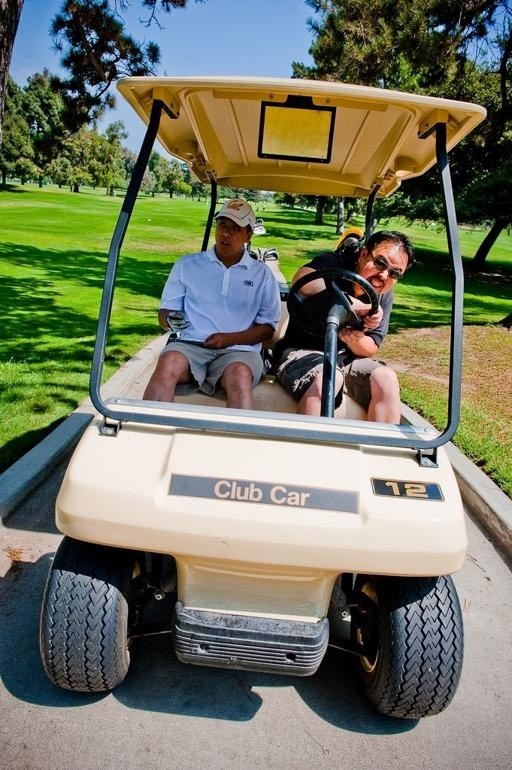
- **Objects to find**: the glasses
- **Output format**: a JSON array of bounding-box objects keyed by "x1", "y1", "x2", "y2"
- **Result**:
[{"x1": 370, "y1": 249, "x2": 400, "y2": 281}]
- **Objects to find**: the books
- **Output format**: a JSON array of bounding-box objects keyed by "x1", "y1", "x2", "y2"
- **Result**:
[{"x1": 170, "y1": 338, "x2": 205, "y2": 347}]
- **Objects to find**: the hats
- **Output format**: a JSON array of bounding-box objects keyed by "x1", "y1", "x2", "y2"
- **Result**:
[{"x1": 214, "y1": 198, "x2": 256, "y2": 234}]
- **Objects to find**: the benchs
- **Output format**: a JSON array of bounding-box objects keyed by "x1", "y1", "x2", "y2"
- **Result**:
[{"x1": 173, "y1": 301, "x2": 368, "y2": 421}]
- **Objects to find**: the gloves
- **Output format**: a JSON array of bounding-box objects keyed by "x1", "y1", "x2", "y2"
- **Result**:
[{"x1": 165, "y1": 309, "x2": 191, "y2": 331}]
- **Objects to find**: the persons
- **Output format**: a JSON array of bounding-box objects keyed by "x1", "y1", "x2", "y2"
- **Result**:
[
  {"x1": 272, "y1": 230, "x2": 415, "y2": 425},
  {"x1": 143, "y1": 198, "x2": 282, "y2": 411}
]
